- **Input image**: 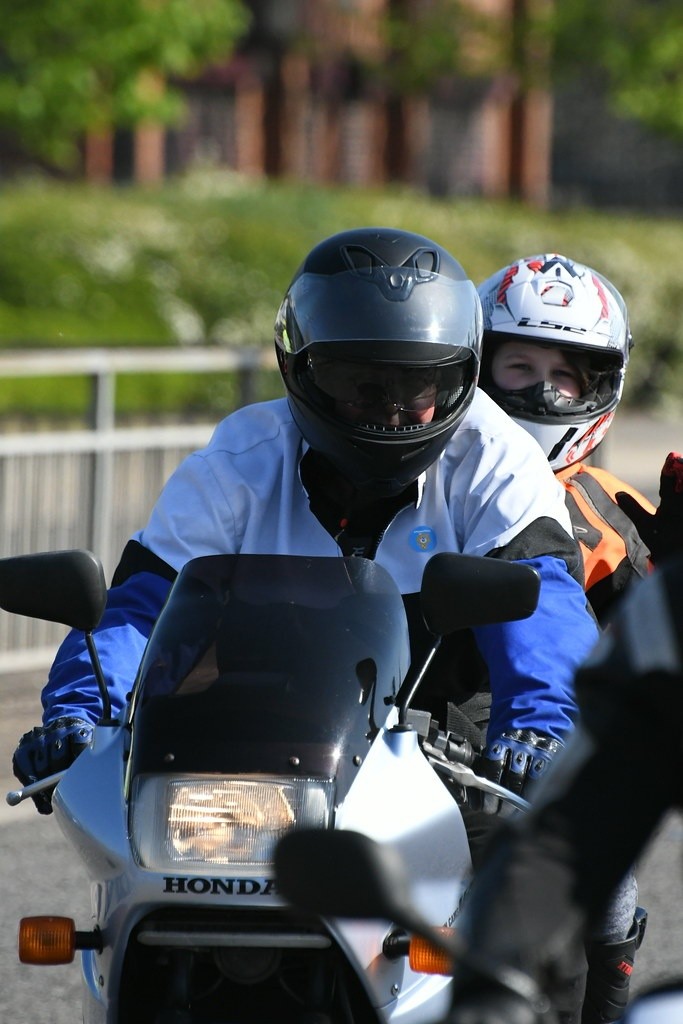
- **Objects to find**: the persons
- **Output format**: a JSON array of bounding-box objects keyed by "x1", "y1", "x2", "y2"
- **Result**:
[
  {"x1": 476, "y1": 250, "x2": 681, "y2": 1014},
  {"x1": 4, "y1": 230, "x2": 609, "y2": 1014}
]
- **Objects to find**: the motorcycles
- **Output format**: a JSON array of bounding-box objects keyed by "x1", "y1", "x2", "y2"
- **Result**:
[{"x1": 1, "y1": 545, "x2": 647, "y2": 1023}]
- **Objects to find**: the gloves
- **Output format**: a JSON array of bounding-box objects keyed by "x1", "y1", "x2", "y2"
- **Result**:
[
  {"x1": 468, "y1": 727, "x2": 566, "y2": 821},
  {"x1": 11, "y1": 718, "x2": 95, "y2": 816},
  {"x1": 613, "y1": 451, "x2": 683, "y2": 567}
]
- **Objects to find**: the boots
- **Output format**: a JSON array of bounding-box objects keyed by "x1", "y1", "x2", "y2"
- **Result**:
[{"x1": 584, "y1": 903, "x2": 646, "y2": 1024}]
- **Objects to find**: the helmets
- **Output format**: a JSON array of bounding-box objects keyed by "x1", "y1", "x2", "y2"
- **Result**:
[
  {"x1": 273, "y1": 228, "x2": 483, "y2": 496},
  {"x1": 476, "y1": 253, "x2": 632, "y2": 474}
]
